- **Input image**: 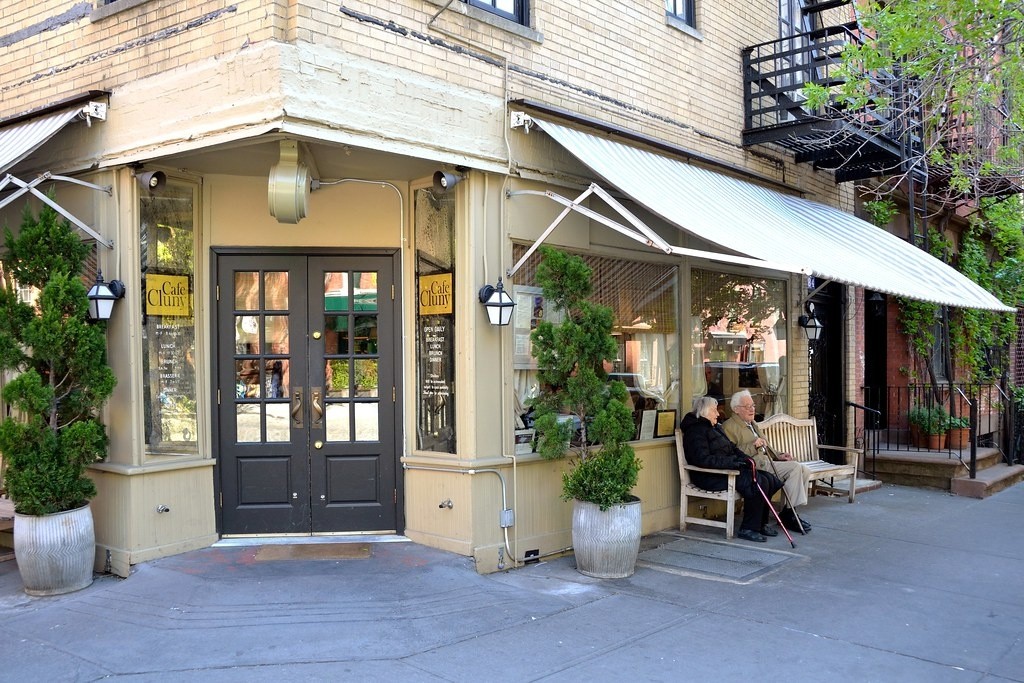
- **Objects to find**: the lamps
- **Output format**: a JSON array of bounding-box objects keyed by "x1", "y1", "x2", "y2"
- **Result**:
[
  {"x1": 479, "y1": 275, "x2": 517, "y2": 326},
  {"x1": 84, "y1": 267, "x2": 125, "y2": 320},
  {"x1": 798, "y1": 308, "x2": 824, "y2": 341}
]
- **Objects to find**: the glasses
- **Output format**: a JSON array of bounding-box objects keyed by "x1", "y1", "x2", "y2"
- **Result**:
[{"x1": 737, "y1": 403, "x2": 755, "y2": 409}]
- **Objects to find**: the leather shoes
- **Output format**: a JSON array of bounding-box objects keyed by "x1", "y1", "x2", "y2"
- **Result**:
[
  {"x1": 778, "y1": 504, "x2": 812, "y2": 531},
  {"x1": 759, "y1": 525, "x2": 778, "y2": 536},
  {"x1": 737, "y1": 528, "x2": 767, "y2": 542}
]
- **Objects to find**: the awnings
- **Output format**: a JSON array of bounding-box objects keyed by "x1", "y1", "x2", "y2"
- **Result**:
[
  {"x1": 506, "y1": 111, "x2": 1019, "y2": 311},
  {"x1": 0, "y1": 101, "x2": 115, "y2": 249}
]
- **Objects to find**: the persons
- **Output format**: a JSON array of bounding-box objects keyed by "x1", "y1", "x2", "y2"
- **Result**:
[
  {"x1": 679, "y1": 396, "x2": 785, "y2": 542},
  {"x1": 723, "y1": 388, "x2": 813, "y2": 532}
]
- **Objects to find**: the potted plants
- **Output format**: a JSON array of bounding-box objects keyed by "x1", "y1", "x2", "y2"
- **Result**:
[
  {"x1": 522, "y1": 237, "x2": 643, "y2": 579},
  {"x1": 906, "y1": 401, "x2": 972, "y2": 451},
  {"x1": 0, "y1": 182, "x2": 119, "y2": 595}
]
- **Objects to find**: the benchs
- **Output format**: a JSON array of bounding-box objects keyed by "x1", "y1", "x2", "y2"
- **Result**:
[{"x1": 674, "y1": 414, "x2": 864, "y2": 541}]
